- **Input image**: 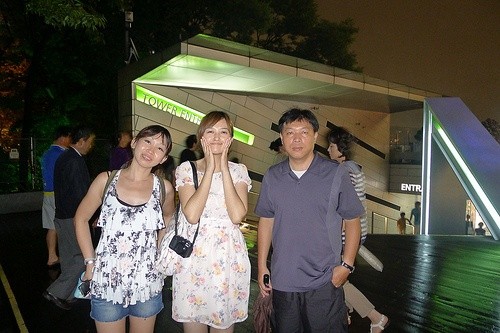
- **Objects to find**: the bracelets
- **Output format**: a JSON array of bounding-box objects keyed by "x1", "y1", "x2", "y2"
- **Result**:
[{"x1": 84, "y1": 258, "x2": 96, "y2": 261}]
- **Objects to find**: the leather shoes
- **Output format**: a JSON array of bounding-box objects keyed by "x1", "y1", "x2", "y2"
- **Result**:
[{"x1": 42, "y1": 290, "x2": 73, "y2": 311}]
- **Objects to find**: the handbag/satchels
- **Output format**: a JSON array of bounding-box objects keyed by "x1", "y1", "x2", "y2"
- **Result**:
[{"x1": 74, "y1": 272, "x2": 92, "y2": 300}]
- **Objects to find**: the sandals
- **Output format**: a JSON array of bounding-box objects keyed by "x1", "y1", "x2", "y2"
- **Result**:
[
  {"x1": 370, "y1": 314, "x2": 391, "y2": 333},
  {"x1": 347, "y1": 317, "x2": 351, "y2": 325}
]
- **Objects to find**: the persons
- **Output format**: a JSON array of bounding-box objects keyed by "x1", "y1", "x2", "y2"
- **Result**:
[
  {"x1": 269, "y1": 137, "x2": 287, "y2": 167},
  {"x1": 254, "y1": 109, "x2": 365, "y2": 332},
  {"x1": 179, "y1": 134, "x2": 198, "y2": 164},
  {"x1": 327, "y1": 128, "x2": 389, "y2": 333},
  {"x1": 409, "y1": 201, "x2": 421, "y2": 235},
  {"x1": 74, "y1": 125, "x2": 174, "y2": 332},
  {"x1": 40, "y1": 127, "x2": 71, "y2": 265},
  {"x1": 475, "y1": 223, "x2": 485, "y2": 235},
  {"x1": 397, "y1": 212, "x2": 412, "y2": 235},
  {"x1": 155, "y1": 111, "x2": 252, "y2": 332},
  {"x1": 43, "y1": 132, "x2": 95, "y2": 310},
  {"x1": 465, "y1": 215, "x2": 473, "y2": 235},
  {"x1": 108, "y1": 130, "x2": 132, "y2": 170}
]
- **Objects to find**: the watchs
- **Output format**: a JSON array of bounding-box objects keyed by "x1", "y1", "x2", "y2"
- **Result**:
[
  {"x1": 84, "y1": 261, "x2": 95, "y2": 265},
  {"x1": 341, "y1": 261, "x2": 355, "y2": 273}
]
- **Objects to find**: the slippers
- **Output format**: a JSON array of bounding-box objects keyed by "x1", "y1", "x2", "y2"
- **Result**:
[{"x1": 46, "y1": 257, "x2": 60, "y2": 267}]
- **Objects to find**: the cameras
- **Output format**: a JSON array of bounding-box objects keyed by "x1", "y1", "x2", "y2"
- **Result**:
[{"x1": 169, "y1": 235, "x2": 193, "y2": 258}]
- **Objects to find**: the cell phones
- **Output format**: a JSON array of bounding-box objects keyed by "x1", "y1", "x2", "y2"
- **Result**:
[{"x1": 78, "y1": 281, "x2": 91, "y2": 297}]
